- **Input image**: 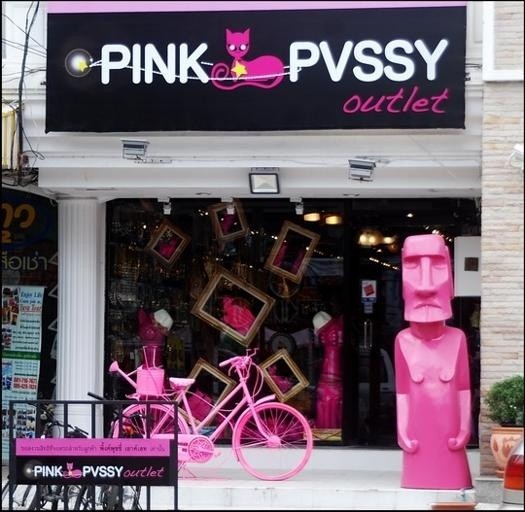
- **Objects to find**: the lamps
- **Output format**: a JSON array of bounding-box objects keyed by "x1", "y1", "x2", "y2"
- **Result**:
[
  {"x1": 120, "y1": 137, "x2": 152, "y2": 165},
  {"x1": 346, "y1": 158, "x2": 376, "y2": 181},
  {"x1": 248, "y1": 166, "x2": 280, "y2": 194}
]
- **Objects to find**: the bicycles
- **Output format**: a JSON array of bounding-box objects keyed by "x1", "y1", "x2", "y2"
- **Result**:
[
  {"x1": 0, "y1": 392, "x2": 155, "y2": 512},
  {"x1": 104, "y1": 347, "x2": 313, "y2": 482}
]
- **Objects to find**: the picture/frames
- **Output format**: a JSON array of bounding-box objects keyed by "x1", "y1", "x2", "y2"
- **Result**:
[{"x1": 147, "y1": 201, "x2": 321, "y2": 426}]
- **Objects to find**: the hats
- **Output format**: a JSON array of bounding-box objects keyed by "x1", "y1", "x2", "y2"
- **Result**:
[
  {"x1": 154, "y1": 308, "x2": 173, "y2": 332},
  {"x1": 311, "y1": 310, "x2": 332, "y2": 335}
]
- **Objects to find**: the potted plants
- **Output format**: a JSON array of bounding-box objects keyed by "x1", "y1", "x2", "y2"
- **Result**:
[{"x1": 482, "y1": 373, "x2": 524, "y2": 480}]
